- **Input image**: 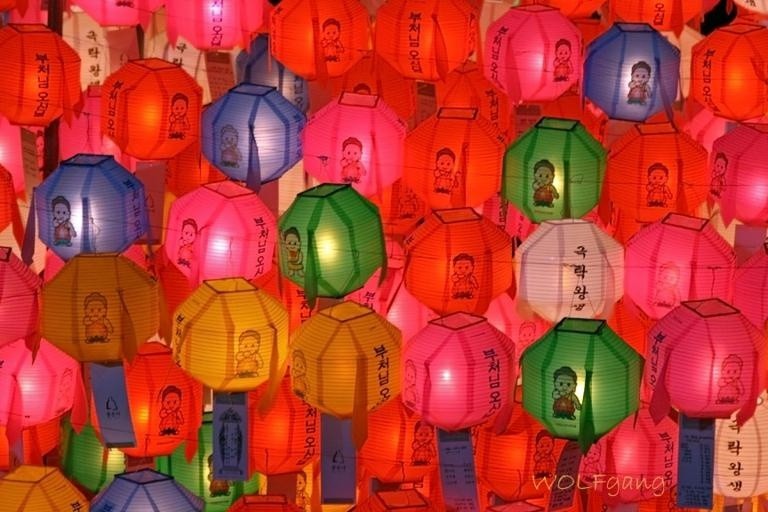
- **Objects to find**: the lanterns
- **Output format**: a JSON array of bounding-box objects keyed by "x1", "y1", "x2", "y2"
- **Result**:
[{"x1": 0, "y1": 0, "x2": 768, "y2": 512}]
